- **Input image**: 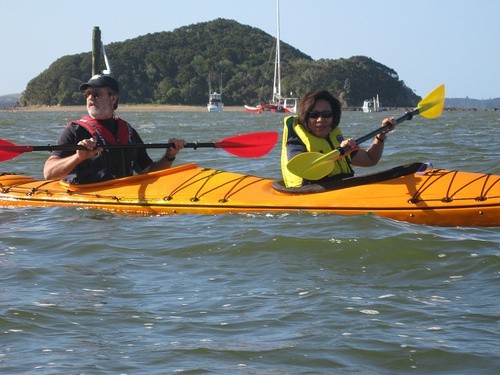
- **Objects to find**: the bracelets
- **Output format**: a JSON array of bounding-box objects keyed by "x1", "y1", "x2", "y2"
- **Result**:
[{"x1": 375, "y1": 136, "x2": 384, "y2": 142}]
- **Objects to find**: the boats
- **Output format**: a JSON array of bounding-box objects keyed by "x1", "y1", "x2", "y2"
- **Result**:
[
  {"x1": 362, "y1": 94, "x2": 382, "y2": 114},
  {"x1": 207, "y1": 70, "x2": 224, "y2": 113},
  {"x1": 0, "y1": 161, "x2": 500, "y2": 229}
]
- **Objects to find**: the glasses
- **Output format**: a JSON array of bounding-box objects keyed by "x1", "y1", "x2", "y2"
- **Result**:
[
  {"x1": 84, "y1": 90, "x2": 112, "y2": 98},
  {"x1": 308, "y1": 111, "x2": 332, "y2": 118}
]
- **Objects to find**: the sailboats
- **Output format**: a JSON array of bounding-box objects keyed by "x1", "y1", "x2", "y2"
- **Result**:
[{"x1": 244, "y1": 0, "x2": 300, "y2": 113}]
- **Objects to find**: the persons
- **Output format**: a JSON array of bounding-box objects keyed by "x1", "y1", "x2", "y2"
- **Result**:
[
  {"x1": 43, "y1": 74, "x2": 183, "y2": 185},
  {"x1": 280, "y1": 85, "x2": 397, "y2": 186}
]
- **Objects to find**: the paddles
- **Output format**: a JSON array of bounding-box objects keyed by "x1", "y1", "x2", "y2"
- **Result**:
[
  {"x1": 286, "y1": 83, "x2": 446, "y2": 180},
  {"x1": 0, "y1": 131, "x2": 279, "y2": 162}
]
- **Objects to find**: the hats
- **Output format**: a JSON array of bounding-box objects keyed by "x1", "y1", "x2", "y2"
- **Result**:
[{"x1": 79, "y1": 74, "x2": 120, "y2": 94}]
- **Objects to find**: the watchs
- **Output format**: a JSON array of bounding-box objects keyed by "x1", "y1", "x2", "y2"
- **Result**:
[{"x1": 165, "y1": 153, "x2": 175, "y2": 161}]
